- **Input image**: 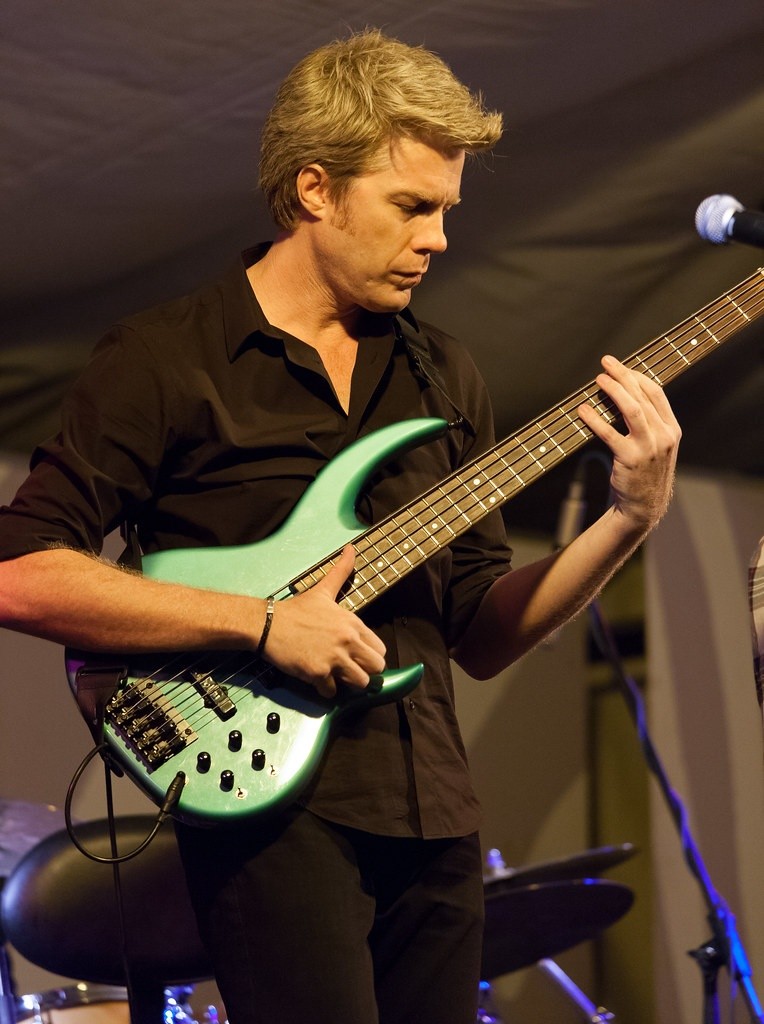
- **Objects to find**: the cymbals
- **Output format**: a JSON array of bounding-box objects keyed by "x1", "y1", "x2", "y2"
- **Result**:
[
  {"x1": 0, "y1": 802, "x2": 84, "y2": 880},
  {"x1": 476, "y1": 840, "x2": 642, "y2": 977}
]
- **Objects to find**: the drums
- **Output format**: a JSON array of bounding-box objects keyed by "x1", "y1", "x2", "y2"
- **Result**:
[{"x1": 12, "y1": 980, "x2": 130, "y2": 1023}]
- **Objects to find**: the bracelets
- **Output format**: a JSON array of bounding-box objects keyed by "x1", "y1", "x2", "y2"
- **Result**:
[{"x1": 254, "y1": 596, "x2": 274, "y2": 662}]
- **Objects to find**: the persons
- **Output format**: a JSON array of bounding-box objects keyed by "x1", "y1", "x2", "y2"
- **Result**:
[{"x1": 0, "y1": 30, "x2": 682, "y2": 1023}]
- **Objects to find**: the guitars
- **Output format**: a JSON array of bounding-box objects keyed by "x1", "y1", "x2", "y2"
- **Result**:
[{"x1": 65, "y1": 264, "x2": 764, "y2": 834}]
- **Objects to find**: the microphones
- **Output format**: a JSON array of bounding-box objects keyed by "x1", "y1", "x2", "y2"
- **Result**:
[
  {"x1": 694, "y1": 194, "x2": 764, "y2": 249},
  {"x1": 554, "y1": 467, "x2": 587, "y2": 551}
]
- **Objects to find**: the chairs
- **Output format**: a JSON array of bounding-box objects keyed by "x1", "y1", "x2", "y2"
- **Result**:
[{"x1": 0, "y1": 813, "x2": 215, "y2": 1024}]
